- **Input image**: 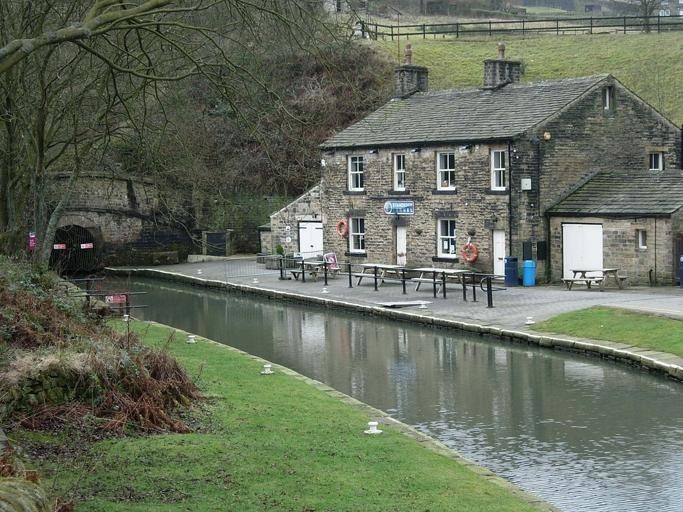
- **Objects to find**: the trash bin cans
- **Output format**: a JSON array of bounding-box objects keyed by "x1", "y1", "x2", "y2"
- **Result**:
[
  {"x1": 522, "y1": 259, "x2": 536, "y2": 287},
  {"x1": 504, "y1": 256, "x2": 518, "y2": 288},
  {"x1": 678, "y1": 254, "x2": 683, "y2": 289}
]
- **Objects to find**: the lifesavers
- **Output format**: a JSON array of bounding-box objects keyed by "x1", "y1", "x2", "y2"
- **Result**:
[
  {"x1": 336, "y1": 220, "x2": 348, "y2": 237},
  {"x1": 462, "y1": 243, "x2": 478, "y2": 262}
]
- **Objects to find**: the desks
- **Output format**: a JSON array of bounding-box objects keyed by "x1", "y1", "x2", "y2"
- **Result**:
[
  {"x1": 569, "y1": 267, "x2": 623, "y2": 292},
  {"x1": 295, "y1": 261, "x2": 325, "y2": 281},
  {"x1": 411, "y1": 267, "x2": 470, "y2": 295},
  {"x1": 357, "y1": 264, "x2": 406, "y2": 288}
]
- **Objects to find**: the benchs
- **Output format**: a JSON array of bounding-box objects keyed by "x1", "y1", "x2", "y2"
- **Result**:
[
  {"x1": 352, "y1": 270, "x2": 408, "y2": 289},
  {"x1": 409, "y1": 275, "x2": 471, "y2": 295},
  {"x1": 290, "y1": 269, "x2": 325, "y2": 282},
  {"x1": 561, "y1": 275, "x2": 630, "y2": 292}
]
